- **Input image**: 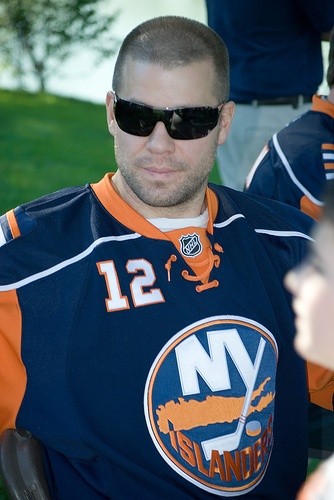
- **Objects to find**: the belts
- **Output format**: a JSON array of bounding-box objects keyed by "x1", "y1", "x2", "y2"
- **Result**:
[{"x1": 227, "y1": 96, "x2": 312, "y2": 105}]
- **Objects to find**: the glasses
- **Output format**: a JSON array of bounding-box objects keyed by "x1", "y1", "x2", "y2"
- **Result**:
[{"x1": 112, "y1": 91, "x2": 231, "y2": 140}]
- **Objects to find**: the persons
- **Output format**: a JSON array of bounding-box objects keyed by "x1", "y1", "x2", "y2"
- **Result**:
[
  {"x1": 243, "y1": 30, "x2": 334, "y2": 222},
  {"x1": 0, "y1": 15, "x2": 334, "y2": 500},
  {"x1": 204, "y1": 0, "x2": 334, "y2": 193},
  {"x1": 282, "y1": 199, "x2": 334, "y2": 500}
]
